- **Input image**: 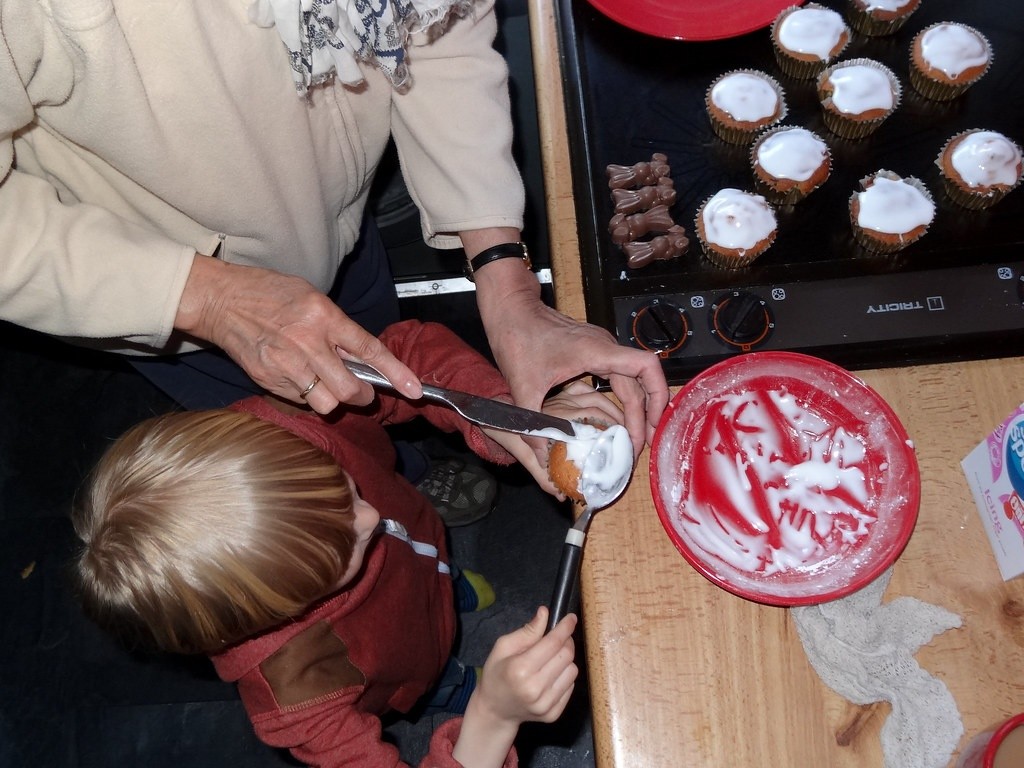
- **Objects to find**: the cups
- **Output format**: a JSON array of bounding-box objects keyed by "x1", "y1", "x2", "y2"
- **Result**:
[{"x1": 956, "y1": 713, "x2": 1023, "y2": 768}]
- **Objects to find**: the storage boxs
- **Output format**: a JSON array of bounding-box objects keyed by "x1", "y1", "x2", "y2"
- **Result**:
[{"x1": 960, "y1": 402, "x2": 1024, "y2": 582}]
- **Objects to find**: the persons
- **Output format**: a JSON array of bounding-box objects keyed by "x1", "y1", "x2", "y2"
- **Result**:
[
  {"x1": 0, "y1": 0, "x2": 669, "y2": 446},
  {"x1": 71, "y1": 318, "x2": 629, "y2": 768}
]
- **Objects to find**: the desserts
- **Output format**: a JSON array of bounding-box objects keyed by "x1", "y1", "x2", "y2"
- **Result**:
[
  {"x1": 545, "y1": 417, "x2": 617, "y2": 508},
  {"x1": 607, "y1": 152, "x2": 693, "y2": 270},
  {"x1": 695, "y1": 0, "x2": 1023, "y2": 270}
]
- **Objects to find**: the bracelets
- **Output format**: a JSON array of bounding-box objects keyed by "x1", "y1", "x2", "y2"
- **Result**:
[{"x1": 462, "y1": 240, "x2": 532, "y2": 282}]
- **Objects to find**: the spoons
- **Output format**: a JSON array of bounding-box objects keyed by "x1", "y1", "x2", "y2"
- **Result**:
[{"x1": 542, "y1": 423, "x2": 635, "y2": 641}]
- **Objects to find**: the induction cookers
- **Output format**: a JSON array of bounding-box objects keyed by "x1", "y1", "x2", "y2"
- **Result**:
[{"x1": 554, "y1": 0, "x2": 1024, "y2": 387}]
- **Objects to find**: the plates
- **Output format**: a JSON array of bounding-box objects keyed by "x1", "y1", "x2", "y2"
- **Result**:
[
  {"x1": 587, "y1": 0, "x2": 811, "y2": 40},
  {"x1": 649, "y1": 350, "x2": 918, "y2": 609}
]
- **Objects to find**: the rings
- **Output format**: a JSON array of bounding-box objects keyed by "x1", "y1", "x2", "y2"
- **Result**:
[{"x1": 300, "y1": 376, "x2": 321, "y2": 399}]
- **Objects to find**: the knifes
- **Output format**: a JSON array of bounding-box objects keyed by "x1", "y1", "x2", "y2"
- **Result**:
[{"x1": 341, "y1": 362, "x2": 577, "y2": 443}]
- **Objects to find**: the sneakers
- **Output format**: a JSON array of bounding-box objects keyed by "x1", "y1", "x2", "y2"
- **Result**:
[{"x1": 410, "y1": 458, "x2": 498, "y2": 527}]
